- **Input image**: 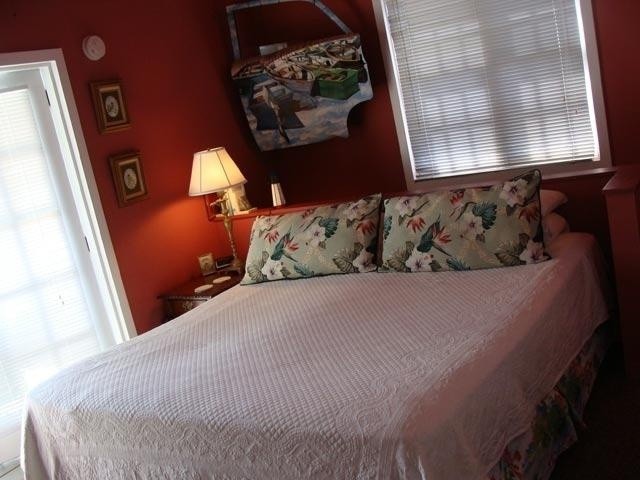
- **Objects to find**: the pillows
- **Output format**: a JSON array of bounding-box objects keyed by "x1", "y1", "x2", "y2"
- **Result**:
[
  {"x1": 241, "y1": 192, "x2": 382, "y2": 284},
  {"x1": 540, "y1": 189, "x2": 565, "y2": 218},
  {"x1": 378, "y1": 168, "x2": 550, "y2": 274},
  {"x1": 542, "y1": 210, "x2": 567, "y2": 248}
]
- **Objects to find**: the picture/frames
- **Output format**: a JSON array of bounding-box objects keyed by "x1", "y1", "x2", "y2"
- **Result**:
[{"x1": 87, "y1": 76, "x2": 151, "y2": 207}]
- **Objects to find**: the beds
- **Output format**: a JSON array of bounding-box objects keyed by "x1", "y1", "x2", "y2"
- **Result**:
[{"x1": 24, "y1": 163, "x2": 617, "y2": 478}]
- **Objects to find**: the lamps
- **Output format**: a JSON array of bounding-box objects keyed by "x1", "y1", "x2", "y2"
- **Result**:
[{"x1": 186, "y1": 145, "x2": 250, "y2": 274}]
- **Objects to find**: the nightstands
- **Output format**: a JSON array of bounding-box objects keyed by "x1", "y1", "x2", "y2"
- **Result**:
[{"x1": 159, "y1": 262, "x2": 241, "y2": 323}]
